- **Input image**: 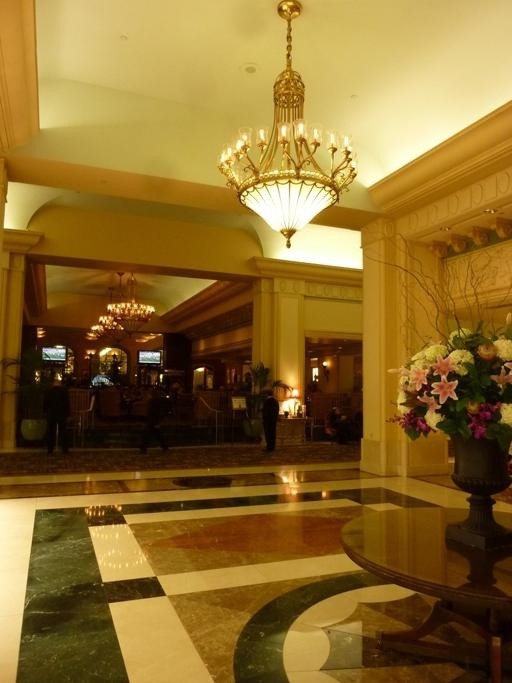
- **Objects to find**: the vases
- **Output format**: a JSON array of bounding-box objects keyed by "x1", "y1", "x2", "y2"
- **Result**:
[{"x1": 445, "y1": 432, "x2": 512, "y2": 551}]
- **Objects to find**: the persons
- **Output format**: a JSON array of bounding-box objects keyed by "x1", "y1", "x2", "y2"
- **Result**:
[
  {"x1": 43, "y1": 381, "x2": 71, "y2": 455},
  {"x1": 262, "y1": 391, "x2": 279, "y2": 453},
  {"x1": 330, "y1": 406, "x2": 350, "y2": 446},
  {"x1": 139, "y1": 391, "x2": 168, "y2": 453}
]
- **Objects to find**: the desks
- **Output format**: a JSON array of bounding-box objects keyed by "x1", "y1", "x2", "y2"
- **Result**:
[{"x1": 339, "y1": 507, "x2": 512, "y2": 682}]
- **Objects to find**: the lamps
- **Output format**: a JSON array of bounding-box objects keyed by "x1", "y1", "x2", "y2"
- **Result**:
[
  {"x1": 218, "y1": 0, "x2": 357, "y2": 249},
  {"x1": 84, "y1": 271, "x2": 155, "y2": 341}
]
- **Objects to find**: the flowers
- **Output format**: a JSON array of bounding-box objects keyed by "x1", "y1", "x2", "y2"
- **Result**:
[{"x1": 386, "y1": 312, "x2": 511, "y2": 450}]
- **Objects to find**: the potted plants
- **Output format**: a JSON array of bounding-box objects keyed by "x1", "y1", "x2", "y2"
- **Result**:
[{"x1": 239, "y1": 361, "x2": 293, "y2": 438}]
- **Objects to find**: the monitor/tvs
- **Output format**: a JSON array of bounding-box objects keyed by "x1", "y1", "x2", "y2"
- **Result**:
[
  {"x1": 136, "y1": 348, "x2": 161, "y2": 365},
  {"x1": 41, "y1": 346, "x2": 68, "y2": 362}
]
